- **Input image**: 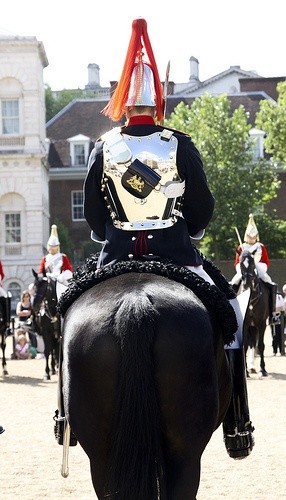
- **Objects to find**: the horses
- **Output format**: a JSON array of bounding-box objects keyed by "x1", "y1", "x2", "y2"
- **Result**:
[
  {"x1": 52, "y1": 260, "x2": 254, "y2": 500},
  {"x1": 0, "y1": 283, "x2": 16, "y2": 378},
  {"x1": 232, "y1": 248, "x2": 275, "y2": 378},
  {"x1": 29, "y1": 267, "x2": 56, "y2": 379}
]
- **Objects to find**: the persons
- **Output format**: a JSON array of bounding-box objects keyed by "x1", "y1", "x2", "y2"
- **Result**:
[
  {"x1": 54, "y1": 62, "x2": 255, "y2": 459},
  {"x1": 0, "y1": 259, "x2": 13, "y2": 335},
  {"x1": 25, "y1": 224, "x2": 73, "y2": 326},
  {"x1": 16, "y1": 284, "x2": 44, "y2": 359},
  {"x1": 229, "y1": 212, "x2": 280, "y2": 325},
  {"x1": 270, "y1": 284, "x2": 286, "y2": 357}
]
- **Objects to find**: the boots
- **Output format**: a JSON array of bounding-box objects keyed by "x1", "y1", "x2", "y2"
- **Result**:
[
  {"x1": 54, "y1": 342, "x2": 77, "y2": 446},
  {"x1": 221, "y1": 347, "x2": 254, "y2": 457}
]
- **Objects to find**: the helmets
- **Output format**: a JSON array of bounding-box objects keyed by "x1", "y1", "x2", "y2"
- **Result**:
[
  {"x1": 98, "y1": 18, "x2": 164, "y2": 123},
  {"x1": 47, "y1": 224, "x2": 60, "y2": 246},
  {"x1": 244, "y1": 213, "x2": 259, "y2": 238}
]
